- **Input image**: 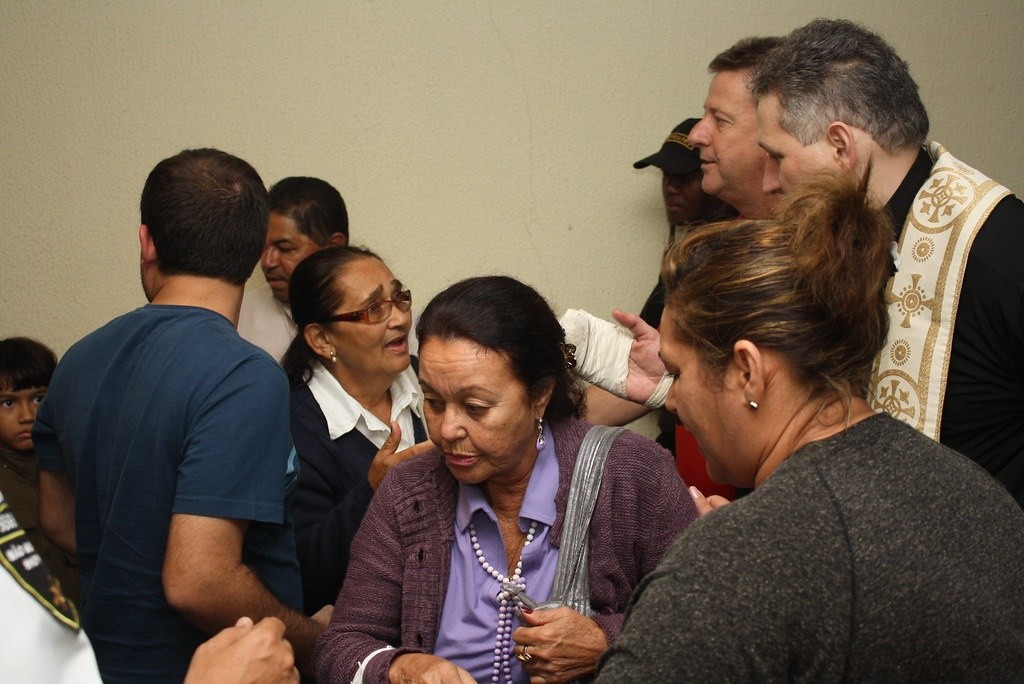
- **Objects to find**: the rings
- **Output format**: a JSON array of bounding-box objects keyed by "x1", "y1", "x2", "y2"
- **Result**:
[{"x1": 515, "y1": 646, "x2": 532, "y2": 662}]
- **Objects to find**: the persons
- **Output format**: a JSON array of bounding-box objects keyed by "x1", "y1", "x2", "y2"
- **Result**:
[
  {"x1": 0, "y1": 495, "x2": 302, "y2": 683},
  {"x1": 568, "y1": 118, "x2": 754, "y2": 499},
  {"x1": 281, "y1": 245, "x2": 652, "y2": 614},
  {"x1": 1, "y1": 334, "x2": 88, "y2": 600},
  {"x1": 30, "y1": 148, "x2": 339, "y2": 683},
  {"x1": 238, "y1": 176, "x2": 349, "y2": 464},
  {"x1": 310, "y1": 274, "x2": 700, "y2": 684},
  {"x1": 590, "y1": 163, "x2": 1024, "y2": 684},
  {"x1": 689, "y1": 36, "x2": 791, "y2": 219},
  {"x1": 554, "y1": 18, "x2": 1024, "y2": 511}
]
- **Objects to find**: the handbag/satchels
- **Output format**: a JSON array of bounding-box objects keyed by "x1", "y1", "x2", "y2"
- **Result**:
[{"x1": 499, "y1": 424, "x2": 629, "y2": 618}]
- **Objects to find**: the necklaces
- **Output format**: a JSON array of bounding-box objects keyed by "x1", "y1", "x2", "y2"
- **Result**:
[{"x1": 465, "y1": 519, "x2": 538, "y2": 684}]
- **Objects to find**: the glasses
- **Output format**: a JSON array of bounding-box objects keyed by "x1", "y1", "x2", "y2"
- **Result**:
[{"x1": 324, "y1": 290, "x2": 412, "y2": 324}]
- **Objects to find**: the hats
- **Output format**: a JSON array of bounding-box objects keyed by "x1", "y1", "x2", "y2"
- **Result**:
[{"x1": 633, "y1": 118, "x2": 704, "y2": 175}]
